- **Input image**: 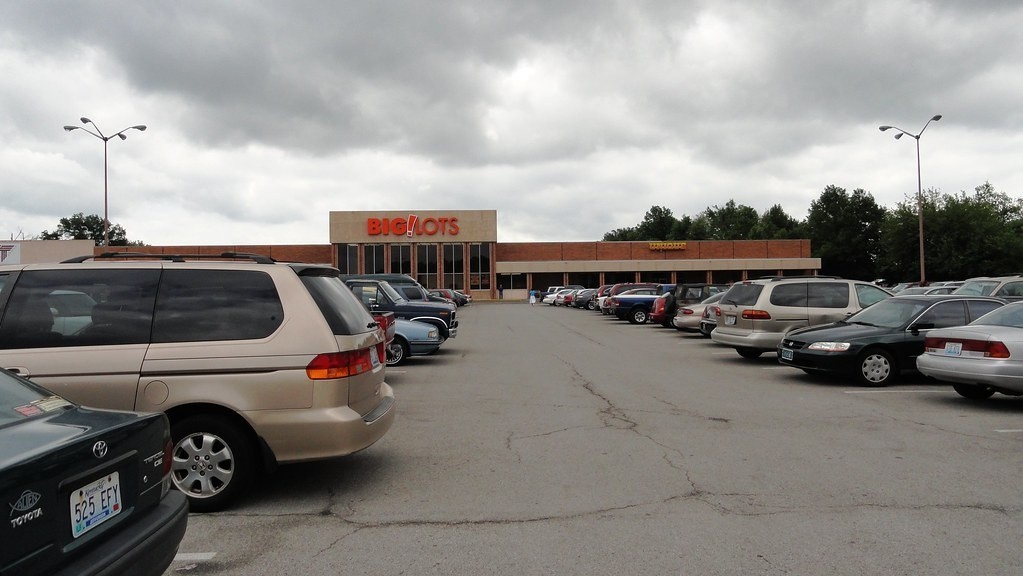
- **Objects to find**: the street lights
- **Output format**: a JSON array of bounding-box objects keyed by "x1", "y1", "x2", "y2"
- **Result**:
[
  {"x1": 879, "y1": 114, "x2": 943, "y2": 286},
  {"x1": 59, "y1": 116, "x2": 147, "y2": 251}
]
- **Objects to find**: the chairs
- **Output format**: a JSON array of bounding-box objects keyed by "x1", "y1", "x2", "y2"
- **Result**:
[
  {"x1": 37, "y1": 301, "x2": 64, "y2": 337},
  {"x1": 81, "y1": 302, "x2": 124, "y2": 338}
]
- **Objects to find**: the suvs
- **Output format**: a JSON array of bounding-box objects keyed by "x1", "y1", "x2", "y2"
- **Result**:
[
  {"x1": 0, "y1": 250, "x2": 400, "y2": 510},
  {"x1": 712, "y1": 276, "x2": 897, "y2": 358},
  {"x1": 342, "y1": 276, "x2": 459, "y2": 356}
]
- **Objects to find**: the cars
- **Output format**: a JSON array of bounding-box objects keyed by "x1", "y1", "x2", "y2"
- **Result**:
[
  {"x1": 777, "y1": 295, "x2": 1009, "y2": 388},
  {"x1": 915, "y1": 300, "x2": 1023, "y2": 399},
  {"x1": 338, "y1": 270, "x2": 473, "y2": 313},
  {"x1": 0, "y1": 367, "x2": 191, "y2": 576},
  {"x1": 386, "y1": 317, "x2": 445, "y2": 368},
  {"x1": 540, "y1": 283, "x2": 728, "y2": 336},
  {"x1": 870, "y1": 274, "x2": 1023, "y2": 301}
]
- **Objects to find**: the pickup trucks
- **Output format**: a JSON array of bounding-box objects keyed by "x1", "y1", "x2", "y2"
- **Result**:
[{"x1": 366, "y1": 306, "x2": 397, "y2": 346}]
